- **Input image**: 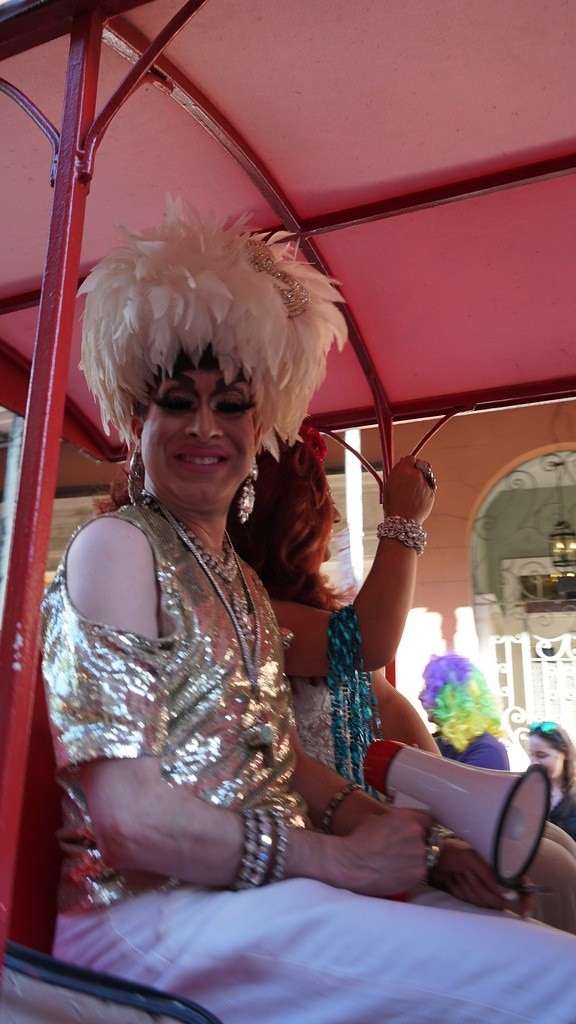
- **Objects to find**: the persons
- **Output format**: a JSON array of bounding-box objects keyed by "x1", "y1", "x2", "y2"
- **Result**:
[
  {"x1": 92, "y1": 422, "x2": 575, "y2": 937},
  {"x1": 415, "y1": 654, "x2": 512, "y2": 776},
  {"x1": 37, "y1": 294, "x2": 576, "y2": 1024},
  {"x1": 525, "y1": 721, "x2": 576, "y2": 842}
]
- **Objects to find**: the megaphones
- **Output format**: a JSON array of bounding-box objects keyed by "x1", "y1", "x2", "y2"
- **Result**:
[{"x1": 363, "y1": 740, "x2": 554, "y2": 886}]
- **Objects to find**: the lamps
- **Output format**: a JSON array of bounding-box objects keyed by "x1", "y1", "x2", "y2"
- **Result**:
[{"x1": 546, "y1": 462, "x2": 576, "y2": 570}]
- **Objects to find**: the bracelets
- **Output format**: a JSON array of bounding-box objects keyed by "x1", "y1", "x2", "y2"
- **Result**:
[
  {"x1": 318, "y1": 781, "x2": 366, "y2": 838},
  {"x1": 376, "y1": 515, "x2": 428, "y2": 555},
  {"x1": 231, "y1": 806, "x2": 258, "y2": 890},
  {"x1": 424, "y1": 823, "x2": 458, "y2": 848},
  {"x1": 268, "y1": 818, "x2": 288, "y2": 886},
  {"x1": 245, "y1": 812, "x2": 273, "y2": 889}
]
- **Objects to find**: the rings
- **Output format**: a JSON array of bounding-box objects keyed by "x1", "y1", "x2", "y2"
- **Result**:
[
  {"x1": 418, "y1": 460, "x2": 439, "y2": 493},
  {"x1": 424, "y1": 842, "x2": 440, "y2": 868}
]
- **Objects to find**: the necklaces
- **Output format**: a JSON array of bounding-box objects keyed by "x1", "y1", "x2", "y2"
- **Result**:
[
  {"x1": 138, "y1": 489, "x2": 278, "y2": 750},
  {"x1": 323, "y1": 606, "x2": 389, "y2": 806}
]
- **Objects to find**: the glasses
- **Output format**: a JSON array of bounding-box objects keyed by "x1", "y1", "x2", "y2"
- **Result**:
[{"x1": 528, "y1": 721, "x2": 562, "y2": 737}]
